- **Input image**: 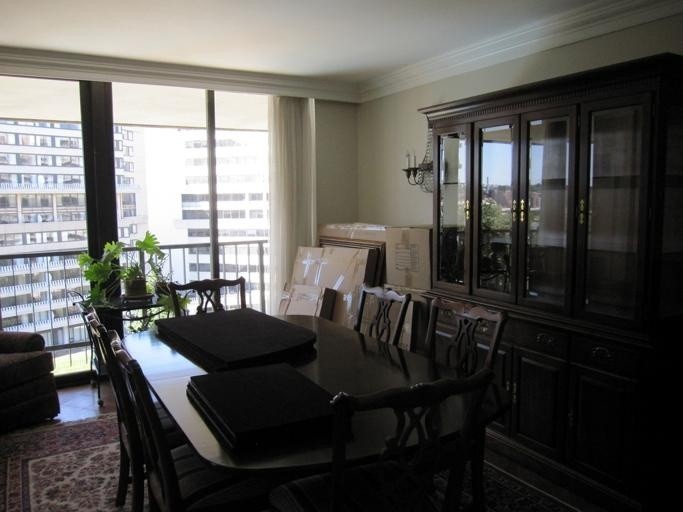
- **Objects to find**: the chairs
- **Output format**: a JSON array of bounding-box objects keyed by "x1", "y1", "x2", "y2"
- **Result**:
[
  {"x1": 109, "y1": 332, "x2": 261, "y2": 510},
  {"x1": 323, "y1": 367, "x2": 495, "y2": 511},
  {"x1": 0, "y1": 321, "x2": 61, "y2": 428},
  {"x1": 168, "y1": 276, "x2": 249, "y2": 317},
  {"x1": 423, "y1": 291, "x2": 505, "y2": 386},
  {"x1": 344, "y1": 280, "x2": 411, "y2": 346},
  {"x1": 79, "y1": 307, "x2": 184, "y2": 512}
]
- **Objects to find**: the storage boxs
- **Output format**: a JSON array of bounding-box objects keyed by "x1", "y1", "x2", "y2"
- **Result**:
[{"x1": 278, "y1": 221, "x2": 434, "y2": 352}]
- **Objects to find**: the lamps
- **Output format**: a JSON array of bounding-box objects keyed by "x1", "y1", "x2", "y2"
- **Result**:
[{"x1": 403, "y1": 148, "x2": 432, "y2": 186}]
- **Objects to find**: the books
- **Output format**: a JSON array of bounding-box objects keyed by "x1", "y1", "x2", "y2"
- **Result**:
[
  {"x1": 189, "y1": 363, "x2": 353, "y2": 437},
  {"x1": 185, "y1": 386, "x2": 352, "y2": 456}
]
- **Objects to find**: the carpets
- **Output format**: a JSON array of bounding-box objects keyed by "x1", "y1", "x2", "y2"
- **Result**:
[{"x1": 0, "y1": 404, "x2": 607, "y2": 512}]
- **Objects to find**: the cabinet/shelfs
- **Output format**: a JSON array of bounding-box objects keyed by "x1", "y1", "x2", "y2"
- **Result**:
[{"x1": 418, "y1": 49, "x2": 683, "y2": 510}]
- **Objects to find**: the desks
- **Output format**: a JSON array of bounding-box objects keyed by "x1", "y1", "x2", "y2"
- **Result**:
[
  {"x1": 84, "y1": 297, "x2": 181, "y2": 412},
  {"x1": 110, "y1": 310, "x2": 512, "y2": 511}
]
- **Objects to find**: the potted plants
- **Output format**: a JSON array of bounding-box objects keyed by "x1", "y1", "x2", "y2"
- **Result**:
[{"x1": 72, "y1": 228, "x2": 192, "y2": 336}]
